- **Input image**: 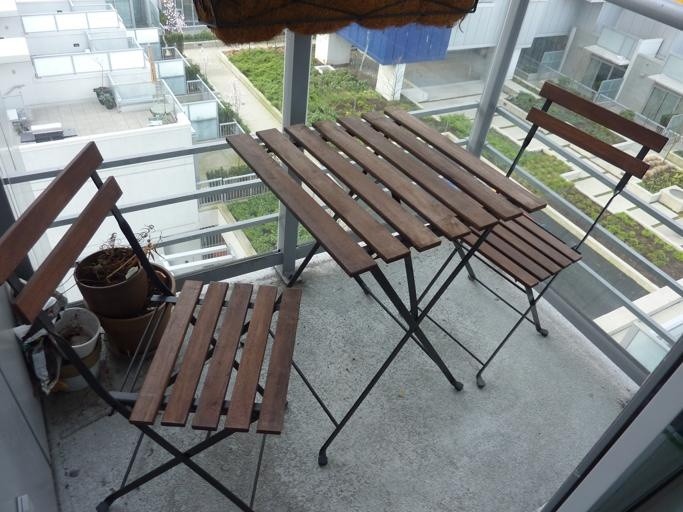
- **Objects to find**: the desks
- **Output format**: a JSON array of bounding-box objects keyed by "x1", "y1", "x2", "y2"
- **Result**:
[{"x1": 226, "y1": 109, "x2": 547, "y2": 466}]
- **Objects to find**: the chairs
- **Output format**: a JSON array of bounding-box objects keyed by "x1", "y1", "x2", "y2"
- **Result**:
[
  {"x1": 407, "y1": 81, "x2": 669, "y2": 389},
  {"x1": 0, "y1": 141, "x2": 303, "y2": 512}
]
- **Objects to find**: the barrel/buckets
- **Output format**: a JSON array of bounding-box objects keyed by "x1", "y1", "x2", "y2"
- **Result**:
[{"x1": 48, "y1": 307, "x2": 107, "y2": 394}]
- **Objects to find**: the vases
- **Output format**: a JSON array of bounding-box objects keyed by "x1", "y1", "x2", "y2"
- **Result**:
[
  {"x1": 72, "y1": 226, "x2": 164, "y2": 317},
  {"x1": 56, "y1": 326, "x2": 103, "y2": 391},
  {"x1": 83, "y1": 262, "x2": 175, "y2": 353},
  {"x1": 52, "y1": 307, "x2": 105, "y2": 360}
]
